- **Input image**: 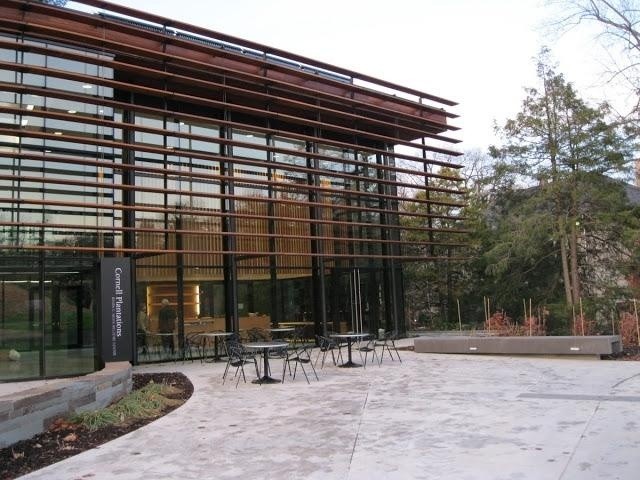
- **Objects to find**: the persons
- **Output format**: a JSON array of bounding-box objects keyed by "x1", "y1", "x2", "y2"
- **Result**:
[
  {"x1": 137, "y1": 302, "x2": 149, "y2": 355},
  {"x1": 159, "y1": 298, "x2": 177, "y2": 354}
]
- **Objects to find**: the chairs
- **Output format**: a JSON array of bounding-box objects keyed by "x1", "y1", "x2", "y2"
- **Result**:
[
  {"x1": 181, "y1": 324, "x2": 402, "y2": 389},
  {"x1": 137, "y1": 328, "x2": 175, "y2": 360}
]
